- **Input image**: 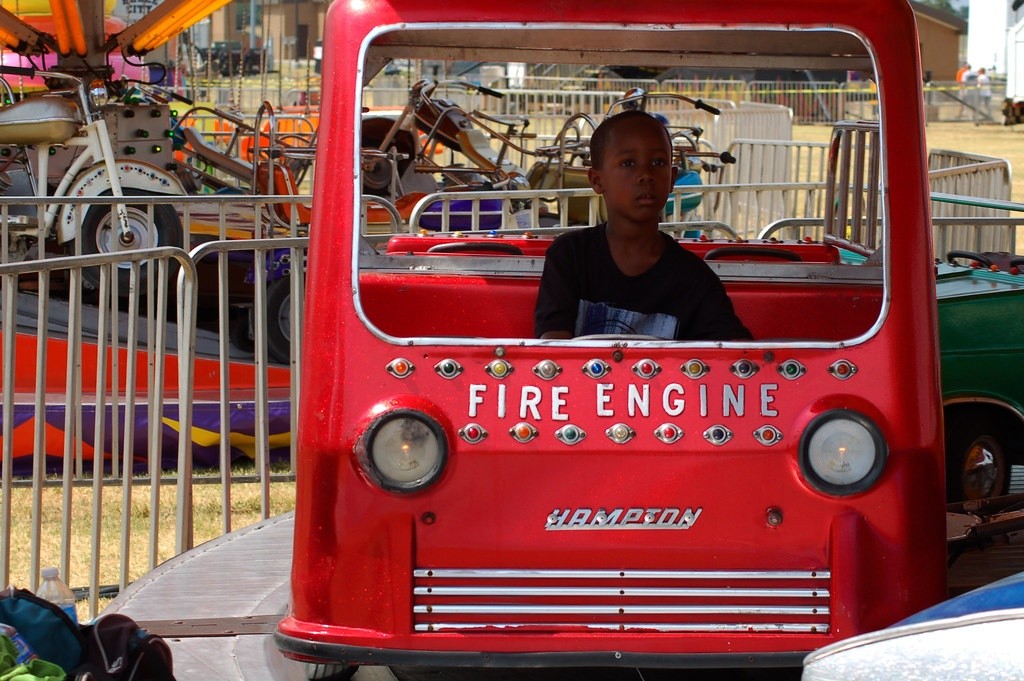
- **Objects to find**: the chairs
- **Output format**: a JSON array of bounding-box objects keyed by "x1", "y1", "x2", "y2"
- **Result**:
[
  {"x1": 556, "y1": 163, "x2": 638, "y2": 224},
  {"x1": 508, "y1": 161, "x2": 563, "y2": 201}
]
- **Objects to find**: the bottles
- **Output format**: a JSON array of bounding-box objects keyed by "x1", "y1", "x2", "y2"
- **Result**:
[
  {"x1": 0, "y1": 622, "x2": 37, "y2": 668},
  {"x1": 34, "y1": 567, "x2": 80, "y2": 633}
]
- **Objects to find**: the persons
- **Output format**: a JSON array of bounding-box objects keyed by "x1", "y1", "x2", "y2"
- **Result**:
[{"x1": 533, "y1": 109, "x2": 755, "y2": 348}]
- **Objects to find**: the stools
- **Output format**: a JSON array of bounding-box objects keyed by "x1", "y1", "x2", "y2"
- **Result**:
[
  {"x1": 360, "y1": 181, "x2": 430, "y2": 223},
  {"x1": 180, "y1": 124, "x2": 259, "y2": 181},
  {"x1": 462, "y1": 119, "x2": 526, "y2": 183}
]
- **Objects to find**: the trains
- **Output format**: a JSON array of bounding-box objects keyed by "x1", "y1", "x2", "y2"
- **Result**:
[{"x1": 260, "y1": 1, "x2": 1024, "y2": 681}]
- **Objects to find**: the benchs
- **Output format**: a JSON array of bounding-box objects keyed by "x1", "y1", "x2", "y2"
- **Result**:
[{"x1": 359, "y1": 270, "x2": 882, "y2": 343}]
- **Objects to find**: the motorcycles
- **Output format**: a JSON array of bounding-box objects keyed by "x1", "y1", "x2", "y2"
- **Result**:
[{"x1": 0, "y1": 0, "x2": 739, "y2": 371}]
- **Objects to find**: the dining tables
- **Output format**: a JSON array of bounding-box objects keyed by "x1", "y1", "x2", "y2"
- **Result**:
[{"x1": 252, "y1": 159, "x2": 316, "y2": 224}]
